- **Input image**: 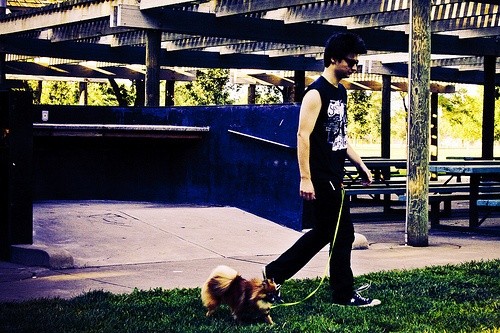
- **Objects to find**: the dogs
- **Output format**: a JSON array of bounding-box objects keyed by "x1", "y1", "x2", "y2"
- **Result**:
[{"x1": 200, "y1": 265, "x2": 282, "y2": 325}]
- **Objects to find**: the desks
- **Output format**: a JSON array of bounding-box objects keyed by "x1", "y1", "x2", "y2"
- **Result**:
[{"x1": 344, "y1": 156, "x2": 500, "y2": 174}]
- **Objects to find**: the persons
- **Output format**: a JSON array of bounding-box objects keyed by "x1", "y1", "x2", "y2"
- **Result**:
[{"x1": 259, "y1": 32, "x2": 381, "y2": 308}]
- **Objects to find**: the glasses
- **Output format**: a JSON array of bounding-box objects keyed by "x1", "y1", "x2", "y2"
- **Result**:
[{"x1": 339, "y1": 56, "x2": 360, "y2": 68}]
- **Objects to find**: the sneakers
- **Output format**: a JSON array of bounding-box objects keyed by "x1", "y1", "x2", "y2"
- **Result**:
[
  {"x1": 255, "y1": 271, "x2": 285, "y2": 304},
  {"x1": 332, "y1": 293, "x2": 381, "y2": 308}
]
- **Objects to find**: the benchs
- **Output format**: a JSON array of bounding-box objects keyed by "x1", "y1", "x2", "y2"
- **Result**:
[{"x1": 341, "y1": 177, "x2": 500, "y2": 235}]
